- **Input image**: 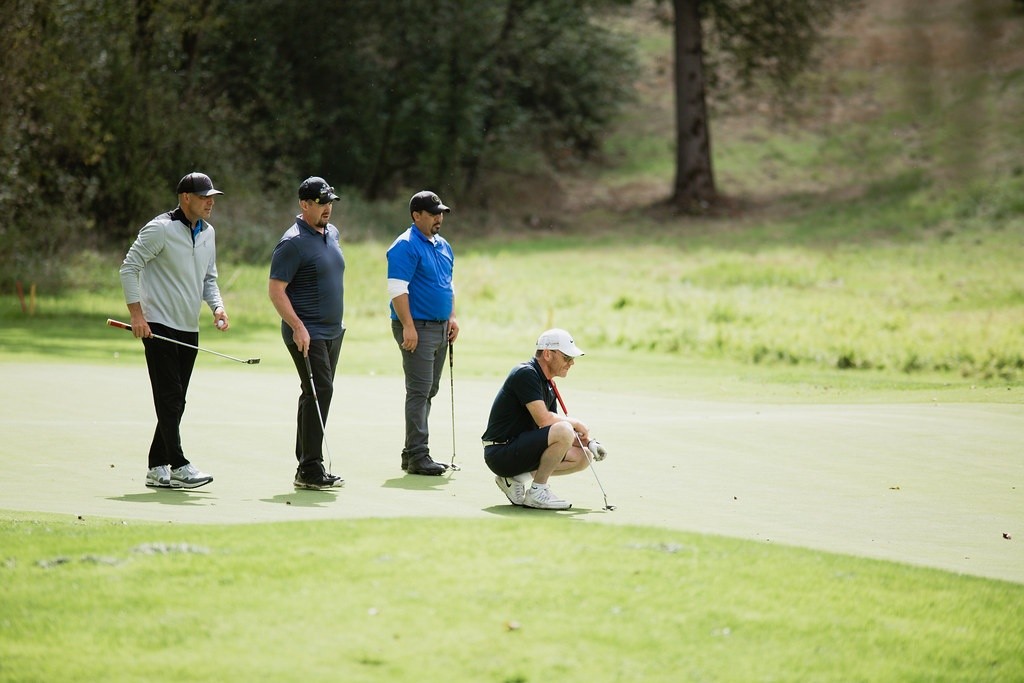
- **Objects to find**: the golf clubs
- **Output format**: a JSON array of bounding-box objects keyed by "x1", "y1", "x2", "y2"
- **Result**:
[
  {"x1": 551, "y1": 380, "x2": 616, "y2": 512},
  {"x1": 106, "y1": 318, "x2": 261, "y2": 365},
  {"x1": 302, "y1": 348, "x2": 345, "y2": 487},
  {"x1": 447, "y1": 331, "x2": 463, "y2": 472}
]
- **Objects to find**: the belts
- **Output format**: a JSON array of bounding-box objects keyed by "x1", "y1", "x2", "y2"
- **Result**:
[{"x1": 483, "y1": 440, "x2": 506, "y2": 446}]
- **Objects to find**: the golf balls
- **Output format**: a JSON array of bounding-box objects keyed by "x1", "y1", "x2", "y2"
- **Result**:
[{"x1": 216, "y1": 318, "x2": 224, "y2": 329}]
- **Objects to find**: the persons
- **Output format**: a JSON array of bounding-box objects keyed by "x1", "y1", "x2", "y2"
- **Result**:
[
  {"x1": 267, "y1": 174, "x2": 346, "y2": 488},
  {"x1": 480, "y1": 327, "x2": 608, "y2": 509},
  {"x1": 385, "y1": 192, "x2": 460, "y2": 474},
  {"x1": 118, "y1": 171, "x2": 230, "y2": 488}
]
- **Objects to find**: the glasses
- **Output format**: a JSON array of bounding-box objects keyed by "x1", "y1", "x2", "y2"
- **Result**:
[{"x1": 553, "y1": 350, "x2": 575, "y2": 362}]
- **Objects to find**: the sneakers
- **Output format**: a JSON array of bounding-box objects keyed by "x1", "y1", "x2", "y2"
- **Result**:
[
  {"x1": 170, "y1": 464, "x2": 213, "y2": 489},
  {"x1": 522, "y1": 484, "x2": 572, "y2": 510},
  {"x1": 293, "y1": 469, "x2": 347, "y2": 486},
  {"x1": 401, "y1": 453, "x2": 448, "y2": 469},
  {"x1": 407, "y1": 455, "x2": 446, "y2": 475},
  {"x1": 495, "y1": 475, "x2": 525, "y2": 506},
  {"x1": 144, "y1": 464, "x2": 170, "y2": 486},
  {"x1": 296, "y1": 469, "x2": 340, "y2": 488}
]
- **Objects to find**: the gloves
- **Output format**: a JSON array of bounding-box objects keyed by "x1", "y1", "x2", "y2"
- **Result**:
[{"x1": 587, "y1": 439, "x2": 607, "y2": 461}]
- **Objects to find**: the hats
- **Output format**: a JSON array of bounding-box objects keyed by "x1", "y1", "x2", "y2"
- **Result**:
[
  {"x1": 299, "y1": 176, "x2": 340, "y2": 204},
  {"x1": 536, "y1": 329, "x2": 585, "y2": 358},
  {"x1": 176, "y1": 172, "x2": 225, "y2": 197},
  {"x1": 409, "y1": 190, "x2": 451, "y2": 215}
]
